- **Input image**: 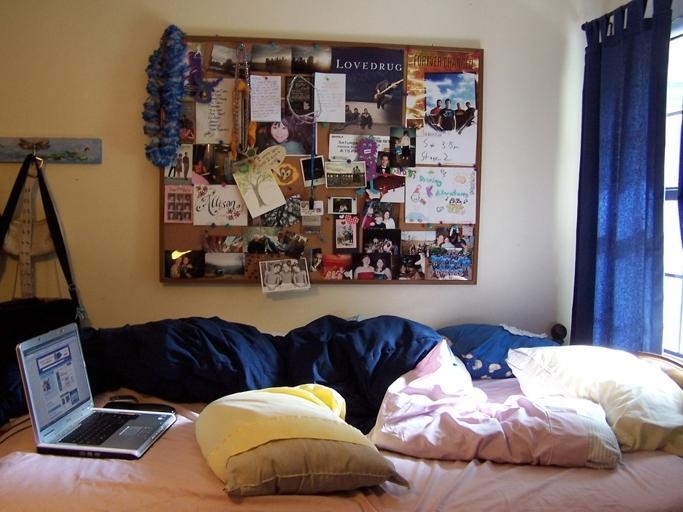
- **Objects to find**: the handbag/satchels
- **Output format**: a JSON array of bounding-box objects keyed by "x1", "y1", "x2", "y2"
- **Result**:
[{"x1": 0, "y1": 296, "x2": 80, "y2": 428}]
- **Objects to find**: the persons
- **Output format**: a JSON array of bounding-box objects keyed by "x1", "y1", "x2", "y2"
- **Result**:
[
  {"x1": 284, "y1": 235, "x2": 305, "y2": 260},
  {"x1": 260, "y1": 114, "x2": 306, "y2": 155},
  {"x1": 324, "y1": 266, "x2": 352, "y2": 280},
  {"x1": 352, "y1": 165, "x2": 361, "y2": 185},
  {"x1": 264, "y1": 261, "x2": 308, "y2": 291},
  {"x1": 369, "y1": 209, "x2": 396, "y2": 229},
  {"x1": 310, "y1": 252, "x2": 322, "y2": 272},
  {"x1": 168, "y1": 152, "x2": 189, "y2": 179},
  {"x1": 178, "y1": 114, "x2": 194, "y2": 139},
  {"x1": 395, "y1": 131, "x2": 411, "y2": 161},
  {"x1": 170, "y1": 256, "x2": 193, "y2": 279},
  {"x1": 376, "y1": 154, "x2": 390, "y2": 174},
  {"x1": 247, "y1": 234, "x2": 265, "y2": 255},
  {"x1": 334, "y1": 105, "x2": 372, "y2": 130},
  {"x1": 430, "y1": 98, "x2": 475, "y2": 131},
  {"x1": 354, "y1": 234, "x2": 472, "y2": 280}
]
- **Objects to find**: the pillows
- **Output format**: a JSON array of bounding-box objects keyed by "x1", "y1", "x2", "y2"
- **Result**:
[
  {"x1": 505, "y1": 345, "x2": 683, "y2": 457},
  {"x1": 371, "y1": 338, "x2": 622, "y2": 472},
  {"x1": 193, "y1": 382, "x2": 409, "y2": 500}
]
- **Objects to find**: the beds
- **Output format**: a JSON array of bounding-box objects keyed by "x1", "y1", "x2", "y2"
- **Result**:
[{"x1": 2, "y1": 324, "x2": 683, "y2": 512}]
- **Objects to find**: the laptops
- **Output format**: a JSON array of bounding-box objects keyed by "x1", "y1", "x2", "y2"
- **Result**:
[{"x1": 16, "y1": 322, "x2": 177, "y2": 460}]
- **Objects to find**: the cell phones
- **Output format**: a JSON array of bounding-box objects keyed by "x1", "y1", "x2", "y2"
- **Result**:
[{"x1": 107, "y1": 395, "x2": 138, "y2": 404}]
